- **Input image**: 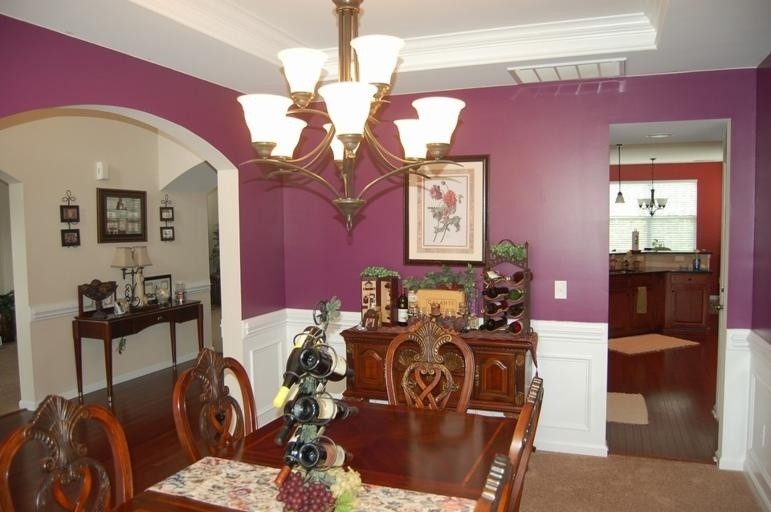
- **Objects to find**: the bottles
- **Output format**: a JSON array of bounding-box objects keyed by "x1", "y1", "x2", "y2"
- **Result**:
[
  {"x1": 478, "y1": 270, "x2": 533, "y2": 334},
  {"x1": 693, "y1": 256, "x2": 701, "y2": 270},
  {"x1": 270, "y1": 315, "x2": 358, "y2": 485},
  {"x1": 632, "y1": 227, "x2": 640, "y2": 251},
  {"x1": 175, "y1": 280, "x2": 186, "y2": 301},
  {"x1": 397, "y1": 284, "x2": 410, "y2": 326},
  {"x1": 408, "y1": 290, "x2": 418, "y2": 319}
]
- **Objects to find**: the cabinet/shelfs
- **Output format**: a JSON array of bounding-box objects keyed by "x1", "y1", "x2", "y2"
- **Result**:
[
  {"x1": 339, "y1": 323, "x2": 538, "y2": 419},
  {"x1": 608, "y1": 272, "x2": 666, "y2": 338},
  {"x1": 666, "y1": 272, "x2": 712, "y2": 332}
]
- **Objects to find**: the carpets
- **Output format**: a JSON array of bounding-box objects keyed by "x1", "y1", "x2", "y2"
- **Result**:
[
  {"x1": 518, "y1": 449, "x2": 764, "y2": 512},
  {"x1": 607, "y1": 392, "x2": 650, "y2": 425},
  {"x1": 608, "y1": 334, "x2": 700, "y2": 355}
]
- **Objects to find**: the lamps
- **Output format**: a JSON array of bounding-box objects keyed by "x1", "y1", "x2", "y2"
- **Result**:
[
  {"x1": 237, "y1": 0, "x2": 466, "y2": 235},
  {"x1": 637, "y1": 158, "x2": 669, "y2": 216},
  {"x1": 615, "y1": 144, "x2": 626, "y2": 203},
  {"x1": 110, "y1": 246, "x2": 153, "y2": 312}
]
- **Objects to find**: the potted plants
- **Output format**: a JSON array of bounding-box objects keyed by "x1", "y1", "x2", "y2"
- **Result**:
[
  {"x1": 209, "y1": 223, "x2": 221, "y2": 304},
  {"x1": 0, "y1": 290, "x2": 15, "y2": 336}
]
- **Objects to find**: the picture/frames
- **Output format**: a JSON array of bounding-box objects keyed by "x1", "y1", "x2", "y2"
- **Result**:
[
  {"x1": 160, "y1": 194, "x2": 175, "y2": 241},
  {"x1": 143, "y1": 274, "x2": 172, "y2": 307},
  {"x1": 97, "y1": 189, "x2": 147, "y2": 243},
  {"x1": 60, "y1": 190, "x2": 80, "y2": 247},
  {"x1": 403, "y1": 155, "x2": 488, "y2": 266}
]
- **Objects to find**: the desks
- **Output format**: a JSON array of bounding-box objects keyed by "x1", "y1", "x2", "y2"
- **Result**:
[
  {"x1": 113, "y1": 400, "x2": 518, "y2": 512},
  {"x1": 71, "y1": 299, "x2": 204, "y2": 411}
]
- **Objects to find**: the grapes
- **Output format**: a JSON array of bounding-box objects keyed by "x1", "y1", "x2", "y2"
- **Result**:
[{"x1": 276, "y1": 470, "x2": 336, "y2": 512}]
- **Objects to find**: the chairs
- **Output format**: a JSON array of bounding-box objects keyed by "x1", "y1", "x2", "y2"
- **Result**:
[
  {"x1": 474, "y1": 374, "x2": 544, "y2": 511},
  {"x1": 384, "y1": 315, "x2": 476, "y2": 413},
  {"x1": 171, "y1": 347, "x2": 257, "y2": 462},
  {"x1": 0, "y1": 394, "x2": 134, "y2": 512}
]
